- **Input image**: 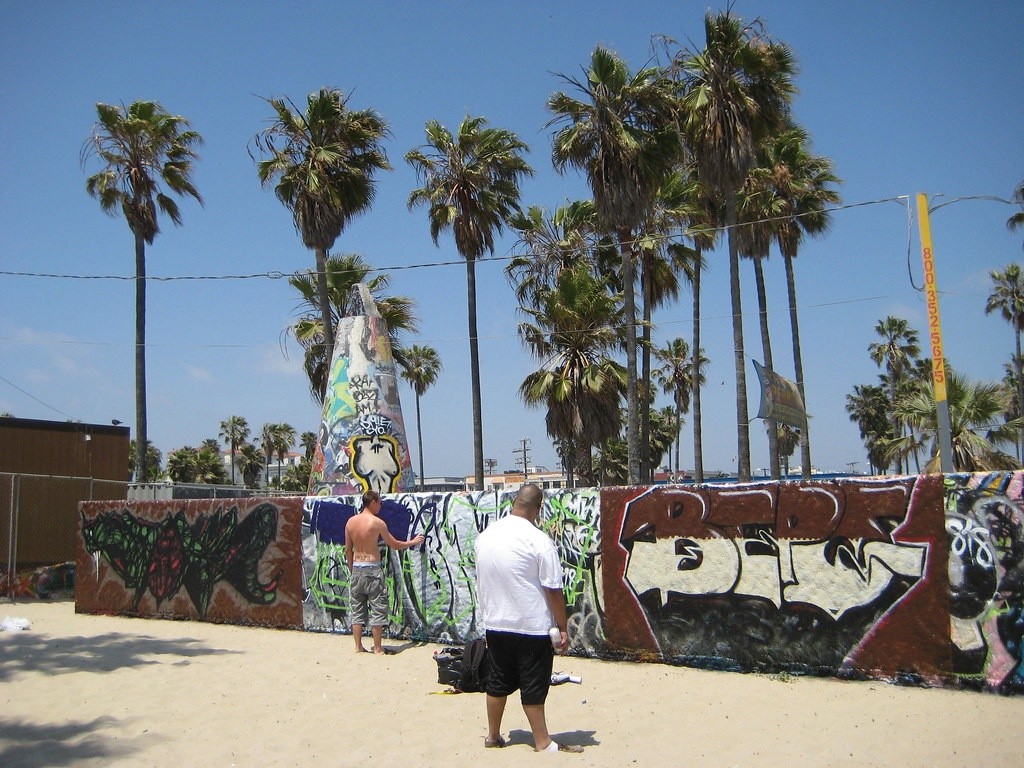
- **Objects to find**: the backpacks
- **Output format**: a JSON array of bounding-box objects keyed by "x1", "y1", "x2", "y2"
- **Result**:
[{"x1": 454, "y1": 639, "x2": 488, "y2": 693}]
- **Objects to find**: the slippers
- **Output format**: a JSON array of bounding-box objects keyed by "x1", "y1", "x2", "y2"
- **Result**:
[
  {"x1": 363, "y1": 648, "x2": 368, "y2": 652},
  {"x1": 384, "y1": 648, "x2": 397, "y2": 655}
]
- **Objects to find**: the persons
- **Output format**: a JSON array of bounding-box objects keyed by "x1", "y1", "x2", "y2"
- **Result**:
[
  {"x1": 345, "y1": 490, "x2": 425, "y2": 655},
  {"x1": 473, "y1": 484, "x2": 584, "y2": 753}
]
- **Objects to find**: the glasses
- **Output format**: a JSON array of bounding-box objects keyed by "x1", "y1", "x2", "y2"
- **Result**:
[{"x1": 377, "y1": 500, "x2": 382, "y2": 505}]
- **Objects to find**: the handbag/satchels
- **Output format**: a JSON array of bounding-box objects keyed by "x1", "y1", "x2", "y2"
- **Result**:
[{"x1": 433, "y1": 648, "x2": 465, "y2": 686}]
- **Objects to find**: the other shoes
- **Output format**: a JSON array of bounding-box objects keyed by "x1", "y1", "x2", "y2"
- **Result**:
[
  {"x1": 553, "y1": 738, "x2": 584, "y2": 753},
  {"x1": 485, "y1": 736, "x2": 507, "y2": 748}
]
